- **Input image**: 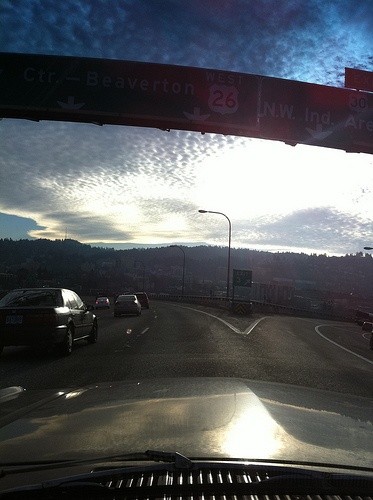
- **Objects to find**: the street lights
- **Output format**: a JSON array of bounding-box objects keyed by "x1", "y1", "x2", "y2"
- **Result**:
[
  {"x1": 170, "y1": 245, "x2": 185, "y2": 297},
  {"x1": 198, "y1": 210, "x2": 232, "y2": 301}
]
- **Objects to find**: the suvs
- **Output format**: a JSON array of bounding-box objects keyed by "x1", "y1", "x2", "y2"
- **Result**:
[{"x1": 133, "y1": 291, "x2": 150, "y2": 309}]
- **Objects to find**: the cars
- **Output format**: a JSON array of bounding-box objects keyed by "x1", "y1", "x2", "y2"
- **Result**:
[
  {"x1": 0, "y1": 287, "x2": 98, "y2": 355},
  {"x1": 95, "y1": 296, "x2": 111, "y2": 310},
  {"x1": 358, "y1": 320, "x2": 373, "y2": 333},
  {"x1": 113, "y1": 295, "x2": 142, "y2": 317}
]
